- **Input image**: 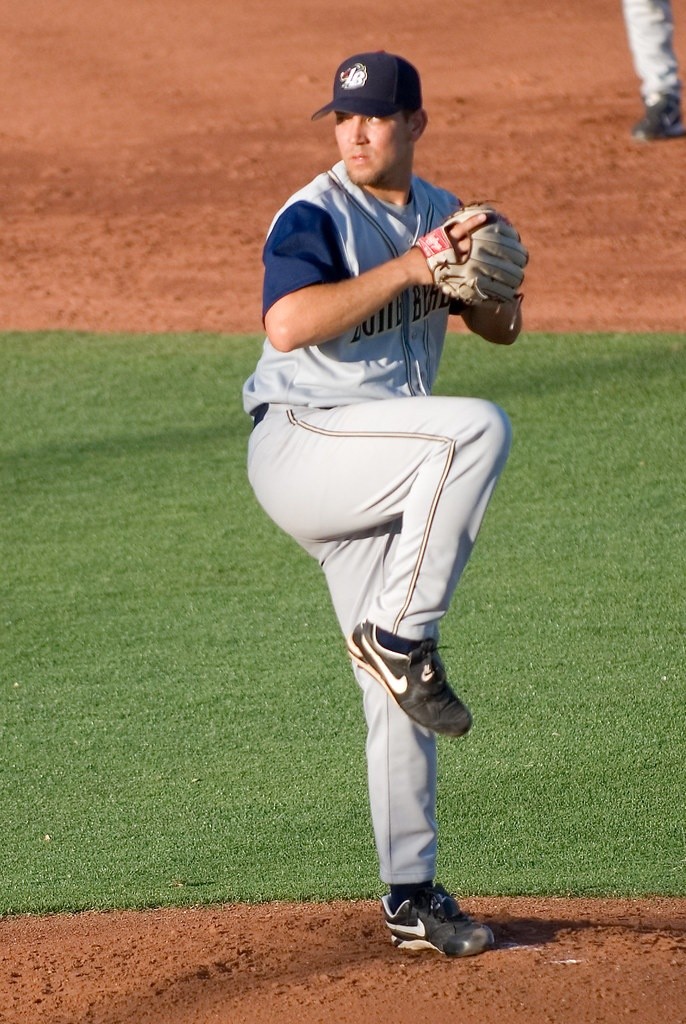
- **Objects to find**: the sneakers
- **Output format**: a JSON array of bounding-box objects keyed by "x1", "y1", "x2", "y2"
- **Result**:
[
  {"x1": 347, "y1": 620, "x2": 472, "y2": 738},
  {"x1": 381, "y1": 882, "x2": 495, "y2": 957},
  {"x1": 631, "y1": 94, "x2": 685, "y2": 139}
]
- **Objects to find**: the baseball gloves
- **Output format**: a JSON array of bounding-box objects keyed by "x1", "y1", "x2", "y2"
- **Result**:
[{"x1": 410, "y1": 199, "x2": 530, "y2": 331}]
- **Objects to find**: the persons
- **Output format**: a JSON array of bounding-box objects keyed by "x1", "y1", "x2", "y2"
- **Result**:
[
  {"x1": 621, "y1": 0, "x2": 684, "y2": 141},
  {"x1": 242, "y1": 51, "x2": 527, "y2": 956}
]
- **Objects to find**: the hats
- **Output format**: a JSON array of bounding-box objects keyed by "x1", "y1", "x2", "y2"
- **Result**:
[{"x1": 310, "y1": 51, "x2": 422, "y2": 118}]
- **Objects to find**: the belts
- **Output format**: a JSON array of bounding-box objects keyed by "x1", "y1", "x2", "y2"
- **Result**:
[{"x1": 253, "y1": 404, "x2": 269, "y2": 428}]
